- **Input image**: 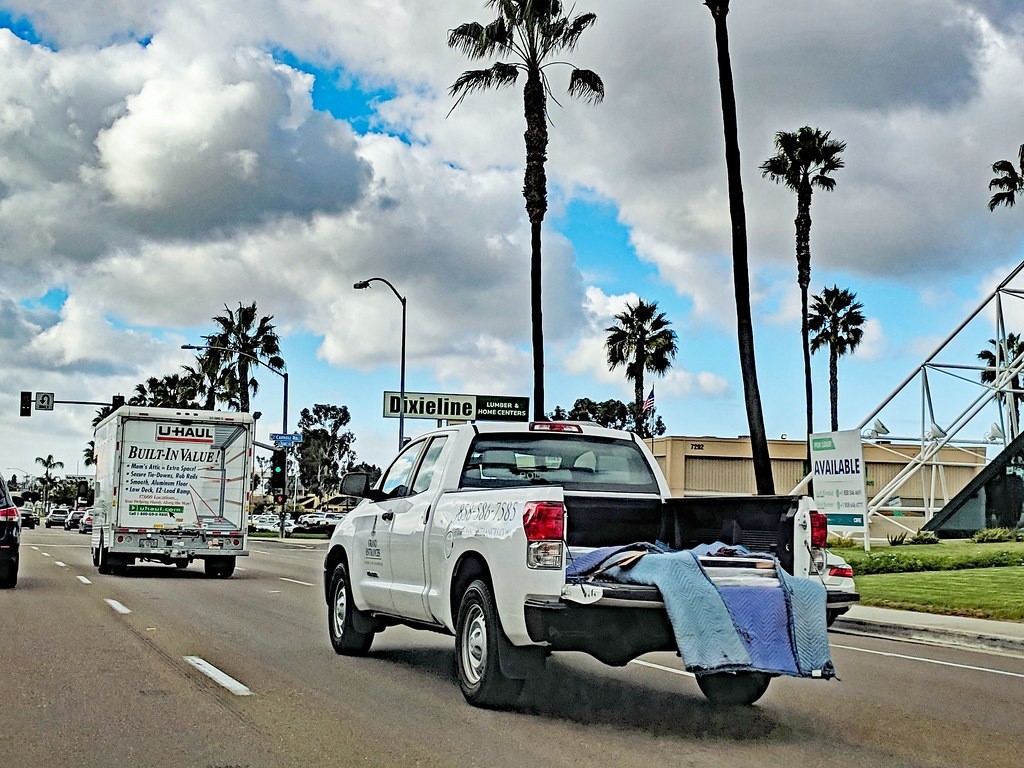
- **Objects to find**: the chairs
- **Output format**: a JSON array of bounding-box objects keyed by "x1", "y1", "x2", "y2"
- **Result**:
[{"x1": 464, "y1": 448, "x2": 636, "y2": 485}]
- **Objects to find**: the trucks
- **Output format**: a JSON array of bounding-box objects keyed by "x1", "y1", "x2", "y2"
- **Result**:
[{"x1": 91, "y1": 405, "x2": 256, "y2": 579}]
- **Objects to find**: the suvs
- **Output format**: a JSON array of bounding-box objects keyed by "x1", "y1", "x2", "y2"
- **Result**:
[
  {"x1": 17, "y1": 508, "x2": 35, "y2": 530},
  {"x1": 45, "y1": 508, "x2": 69, "y2": 528},
  {"x1": 0, "y1": 472, "x2": 25, "y2": 590},
  {"x1": 79, "y1": 509, "x2": 94, "y2": 534},
  {"x1": 64, "y1": 511, "x2": 84, "y2": 530}
]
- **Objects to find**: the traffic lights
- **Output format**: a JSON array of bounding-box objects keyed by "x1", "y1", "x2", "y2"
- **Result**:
[
  {"x1": 271, "y1": 449, "x2": 287, "y2": 506},
  {"x1": 20, "y1": 391, "x2": 32, "y2": 417}
]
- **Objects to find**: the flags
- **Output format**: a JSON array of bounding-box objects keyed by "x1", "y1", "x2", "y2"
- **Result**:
[{"x1": 642, "y1": 388, "x2": 655, "y2": 413}]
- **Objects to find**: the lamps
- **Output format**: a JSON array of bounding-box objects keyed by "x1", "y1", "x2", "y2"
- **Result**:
[
  {"x1": 924, "y1": 422, "x2": 948, "y2": 441},
  {"x1": 861, "y1": 418, "x2": 891, "y2": 439},
  {"x1": 983, "y1": 422, "x2": 1006, "y2": 442}
]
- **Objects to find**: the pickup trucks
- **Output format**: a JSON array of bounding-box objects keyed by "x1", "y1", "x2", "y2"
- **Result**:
[{"x1": 322, "y1": 421, "x2": 831, "y2": 707}]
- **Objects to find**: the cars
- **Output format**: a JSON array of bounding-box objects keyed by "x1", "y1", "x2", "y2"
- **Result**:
[
  {"x1": 821, "y1": 548, "x2": 861, "y2": 629},
  {"x1": 246, "y1": 512, "x2": 348, "y2": 533},
  {"x1": 33, "y1": 513, "x2": 41, "y2": 526}
]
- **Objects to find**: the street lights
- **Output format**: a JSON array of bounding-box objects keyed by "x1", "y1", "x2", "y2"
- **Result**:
[
  {"x1": 353, "y1": 276, "x2": 407, "y2": 452},
  {"x1": 181, "y1": 343, "x2": 289, "y2": 433}
]
